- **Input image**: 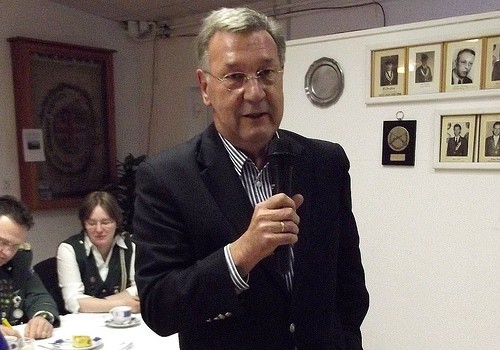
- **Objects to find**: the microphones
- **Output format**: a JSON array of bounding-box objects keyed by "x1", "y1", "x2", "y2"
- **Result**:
[{"x1": 267, "y1": 138, "x2": 295, "y2": 273}]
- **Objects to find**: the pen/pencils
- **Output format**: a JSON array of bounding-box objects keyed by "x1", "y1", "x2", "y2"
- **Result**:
[{"x1": 1, "y1": 318, "x2": 12, "y2": 329}]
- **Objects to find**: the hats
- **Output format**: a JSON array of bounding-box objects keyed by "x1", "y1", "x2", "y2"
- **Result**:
[{"x1": 385, "y1": 59, "x2": 393, "y2": 64}]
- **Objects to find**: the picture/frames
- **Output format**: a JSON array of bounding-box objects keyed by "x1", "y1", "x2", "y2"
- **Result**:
[
  {"x1": 441, "y1": 37, "x2": 484, "y2": 92},
  {"x1": 372, "y1": 46, "x2": 407, "y2": 96},
  {"x1": 477, "y1": 113, "x2": 500, "y2": 162},
  {"x1": 7, "y1": 36, "x2": 119, "y2": 210},
  {"x1": 406, "y1": 42, "x2": 444, "y2": 95},
  {"x1": 482, "y1": 35, "x2": 500, "y2": 89},
  {"x1": 439, "y1": 114, "x2": 478, "y2": 162}
]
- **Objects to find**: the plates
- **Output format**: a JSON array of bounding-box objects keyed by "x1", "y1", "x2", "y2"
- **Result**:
[
  {"x1": 106, "y1": 317, "x2": 140, "y2": 328},
  {"x1": 303, "y1": 57, "x2": 344, "y2": 107},
  {"x1": 38, "y1": 334, "x2": 104, "y2": 350}
]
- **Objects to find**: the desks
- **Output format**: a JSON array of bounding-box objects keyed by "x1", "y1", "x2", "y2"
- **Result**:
[{"x1": 0, "y1": 313, "x2": 180, "y2": 350}]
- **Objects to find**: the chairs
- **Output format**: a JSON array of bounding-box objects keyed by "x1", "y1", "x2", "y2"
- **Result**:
[{"x1": 34, "y1": 257, "x2": 70, "y2": 315}]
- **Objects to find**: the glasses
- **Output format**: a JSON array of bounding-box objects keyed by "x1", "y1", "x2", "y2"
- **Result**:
[
  {"x1": 202, "y1": 69, "x2": 284, "y2": 91},
  {"x1": 0, "y1": 241, "x2": 25, "y2": 251},
  {"x1": 83, "y1": 219, "x2": 114, "y2": 228}
]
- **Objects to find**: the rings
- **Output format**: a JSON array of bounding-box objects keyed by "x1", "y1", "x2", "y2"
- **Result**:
[
  {"x1": 42, "y1": 331, "x2": 47, "y2": 335},
  {"x1": 280, "y1": 221, "x2": 285, "y2": 233}
]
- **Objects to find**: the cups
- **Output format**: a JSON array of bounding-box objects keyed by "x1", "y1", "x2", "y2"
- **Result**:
[{"x1": 109, "y1": 306, "x2": 132, "y2": 325}]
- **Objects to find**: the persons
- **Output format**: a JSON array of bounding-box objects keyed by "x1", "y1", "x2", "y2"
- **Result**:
[
  {"x1": 134, "y1": 4, "x2": 370, "y2": 350},
  {"x1": 0, "y1": 194, "x2": 60, "y2": 342},
  {"x1": 416, "y1": 54, "x2": 432, "y2": 82},
  {"x1": 492, "y1": 47, "x2": 500, "y2": 81},
  {"x1": 452, "y1": 48, "x2": 475, "y2": 84},
  {"x1": 485, "y1": 121, "x2": 500, "y2": 157},
  {"x1": 447, "y1": 124, "x2": 468, "y2": 156},
  {"x1": 57, "y1": 191, "x2": 140, "y2": 313},
  {"x1": 381, "y1": 59, "x2": 398, "y2": 86}
]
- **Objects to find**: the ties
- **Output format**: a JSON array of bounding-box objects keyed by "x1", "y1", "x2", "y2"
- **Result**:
[
  {"x1": 458, "y1": 80, "x2": 462, "y2": 84},
  {"x1": 455, "y1": 138, "x2": 457, "y2": 143}
]
